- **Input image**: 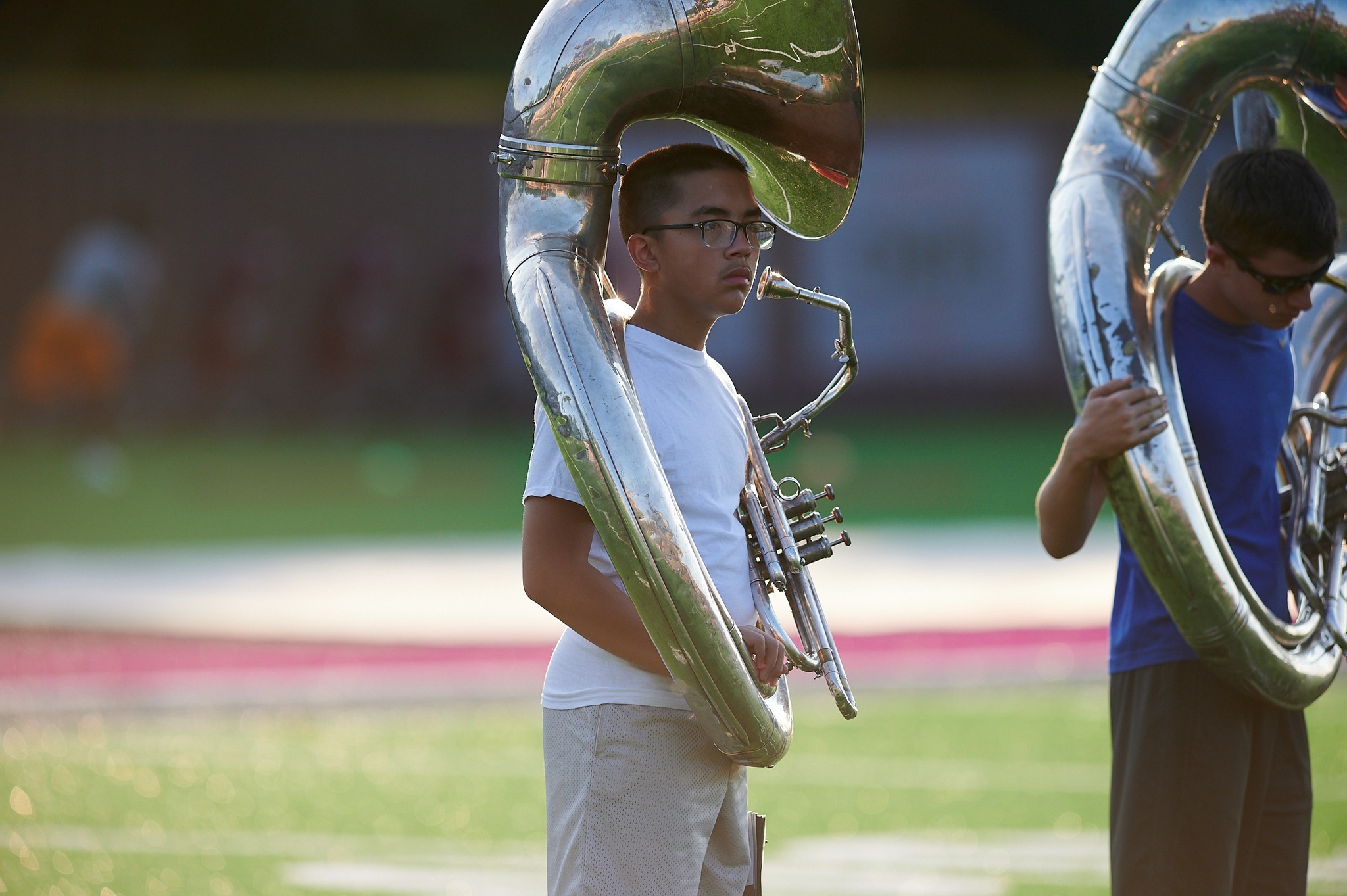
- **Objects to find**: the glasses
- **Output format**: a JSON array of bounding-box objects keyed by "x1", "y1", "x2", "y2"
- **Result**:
[
  {"x1": 641, "y1": 218, "x2": 779, "y2": 251},
  {"x1": 1215, "y1": 234, "x2": 1335, "y2": 297}
]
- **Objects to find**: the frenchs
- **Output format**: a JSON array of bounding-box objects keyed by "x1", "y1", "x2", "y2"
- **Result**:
[
  {"x1": 487, "y1": 0, "x2": 868, "y2": 770},
  {"x1": 1045, "y1": 0, "x2": 1346, "y2": 712}
]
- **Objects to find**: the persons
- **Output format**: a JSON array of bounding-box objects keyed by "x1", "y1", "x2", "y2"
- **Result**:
[
  {"x1": 1035, "y1": 147, "x2": 1337, "y2": 896},
  {"x1": 522, "y1": 143, "x2": 790, "y2": 896},
  {"x1": 18, "y1": 213, "x2": 163, "y2": 442}
]
